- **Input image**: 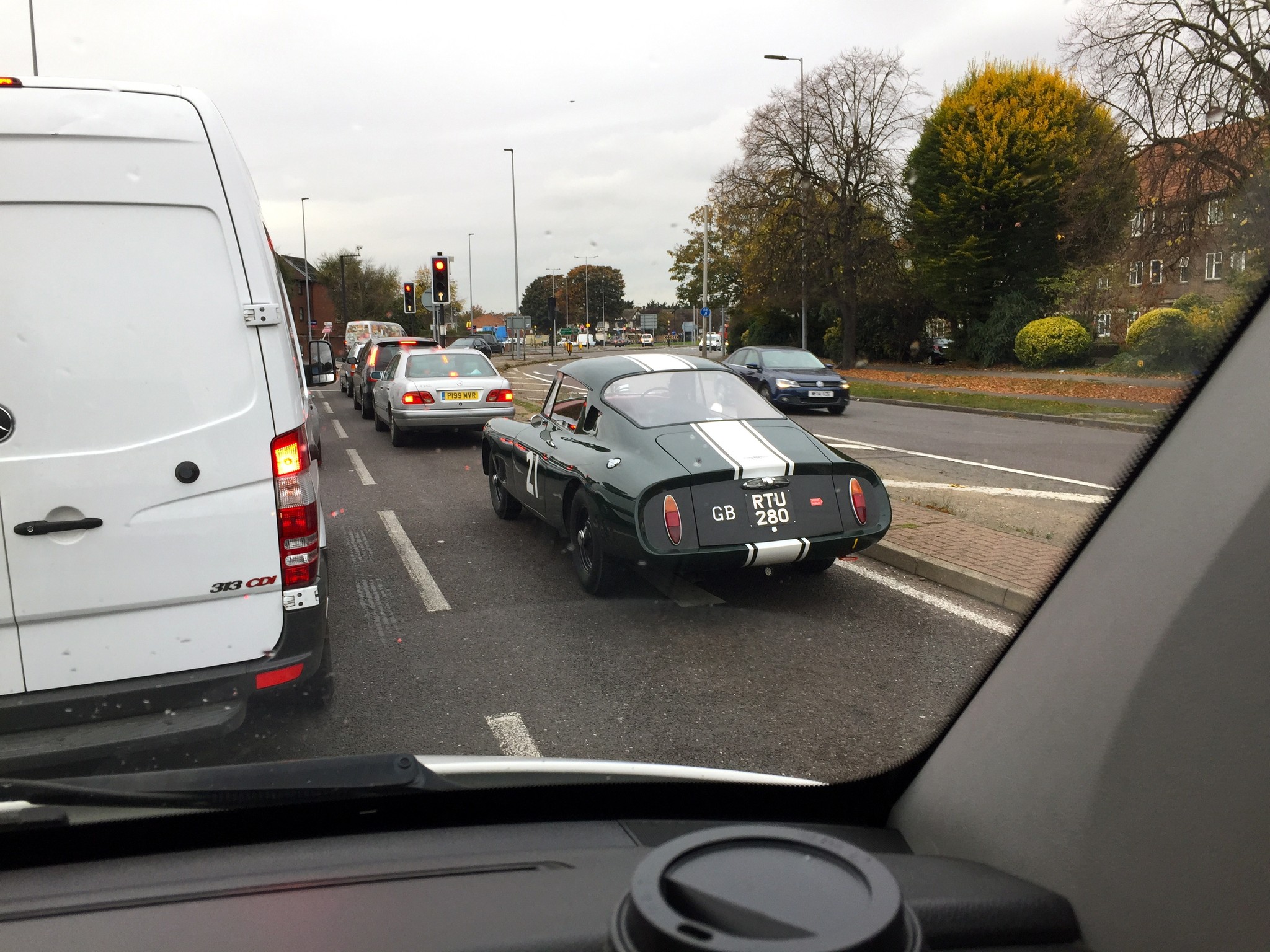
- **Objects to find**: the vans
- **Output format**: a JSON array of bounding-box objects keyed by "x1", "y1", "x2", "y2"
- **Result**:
[
  {"x1": 641, "y1": 333, "x2": 654, "y2": 348},
  {"x1": 344, "y1": 320, "x2": 407, "y2": 348},
  {"x1": 0, "y1": 0, "x2": 337, "y2": 777},
  {"x1": 577, "y1": 334, "x2": 597, "y2": 346}
]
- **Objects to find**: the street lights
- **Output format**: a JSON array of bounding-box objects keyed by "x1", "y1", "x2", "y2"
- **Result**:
[
  {"x1": 340, "y1": 244, "x2": 364, "y2": 336},
  {"x1": 573, "y1": 255, "x2": 601, "y2": 349},
  {"x1": 545, "y1": 268, "x2": 561, "y2": 346},
  {"x1": 504, "y1": 147, "x2": 521, "y2": 361},
  {"x1": 468, "y1": 232, "x2": 475, "y2": 336},
  {"x1": 764, "y1": 53, "x2": 811, "y2": 356}
]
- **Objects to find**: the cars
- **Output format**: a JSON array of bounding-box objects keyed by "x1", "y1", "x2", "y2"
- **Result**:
[
  {"x1": 338, "y1": 337, "x2": 443, "y2": 421},
  {"x1": 447, "y1": 338, "x2": 492, "y2": 361},
  {"x1": 614, "y1": 339, "x2": 628, "y2": 347},
  {"x1": 557, "y1": 339, "x2": 577, "y2": 347},
  {"x1": 722, "y1": 343, "x2": 851, "y2": 418},
  {"x1": 371, "y1": 347, "x2": 514, "y2": 447},
  {"x1": 481, "y1": 353, "x2": 893, "y2": 602}
]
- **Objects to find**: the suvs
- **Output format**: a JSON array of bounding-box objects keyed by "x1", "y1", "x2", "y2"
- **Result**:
[
  {"x1": 699, "y1": 333, "x2": 722, "y2": 351},
  {"x1": 469, "y1": 334, "x2": 506, "y2": 354}
]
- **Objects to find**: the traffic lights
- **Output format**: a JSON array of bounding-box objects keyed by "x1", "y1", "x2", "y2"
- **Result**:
[
  {"x1": 431, "y1": 256, "x2": 451, "y2": 305},
  {"x1": 403, "y1": 282, "x2": 416, "y2": 315}
]
